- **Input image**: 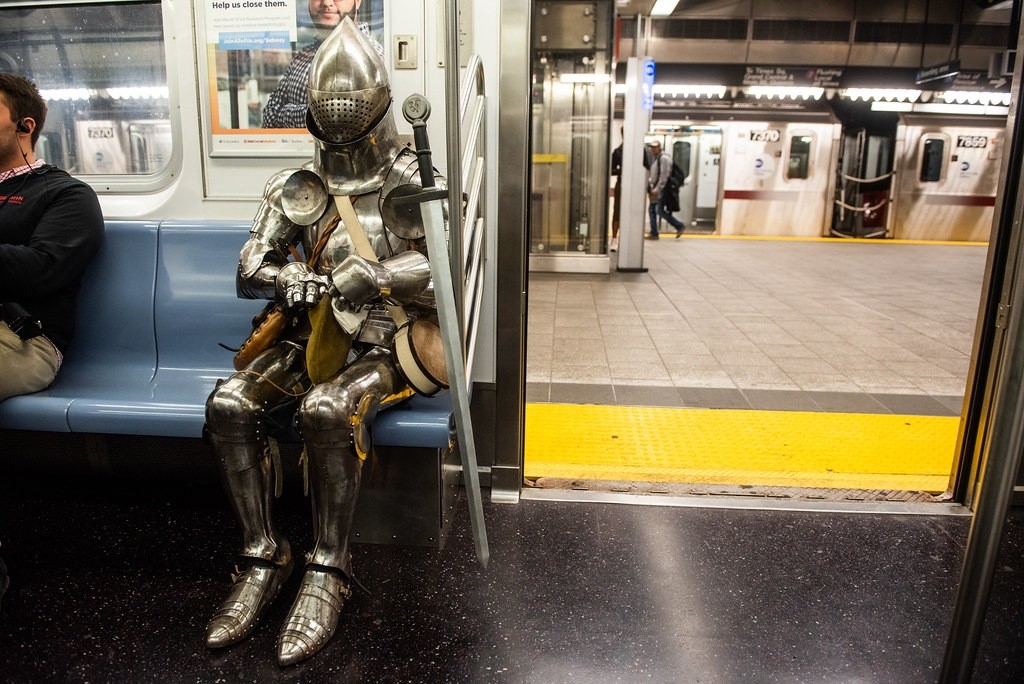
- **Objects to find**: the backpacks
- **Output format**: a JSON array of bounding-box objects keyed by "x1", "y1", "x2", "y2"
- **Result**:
[{"x1": 656, "y1": 152, "x2": 685, "y2": 205}]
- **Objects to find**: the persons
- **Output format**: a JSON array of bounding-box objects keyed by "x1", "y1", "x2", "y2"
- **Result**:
[
  {"x1": 610, "y1": 126, "x2": 650, "y2": 252},
  {"x1": 644, "y1": 141, "x2": 686, "y2": 240},
  {"x1": 262, "y1": 1, "x2": 384, "y2": 129},
  {"x1": 202, "y1": 15, "x2": 451, "y2": 664},
  {"x1": 0, "y1": 70, "x2": 107, "y2": 400}
]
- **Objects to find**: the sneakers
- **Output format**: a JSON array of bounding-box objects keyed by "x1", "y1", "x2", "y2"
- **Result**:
[{"x1": 610, "y1": 237, "x2": 618, "y2": 252}]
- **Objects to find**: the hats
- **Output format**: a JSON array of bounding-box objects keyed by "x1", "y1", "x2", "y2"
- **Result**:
[{"x1": 648, "y1": 139, "x2": 660, "y2": 147}]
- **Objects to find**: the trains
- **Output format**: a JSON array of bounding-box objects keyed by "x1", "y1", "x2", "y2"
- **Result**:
[{"x1": 578, "y1": 113, "x2": 1010, "y2": 241}]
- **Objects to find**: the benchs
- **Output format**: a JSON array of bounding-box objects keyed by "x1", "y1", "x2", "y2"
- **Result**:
[{"x1": 0, "y1": 218, "x2": 474, "y2": 551}]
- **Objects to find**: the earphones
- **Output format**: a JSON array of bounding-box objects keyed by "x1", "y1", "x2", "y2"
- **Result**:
[{"x1": 17, "y1": 121, "x2": 30, "y2": 133}]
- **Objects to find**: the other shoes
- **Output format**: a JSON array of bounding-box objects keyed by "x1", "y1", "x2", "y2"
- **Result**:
[
  {"x1": 643, "y1": 233, "x2": 659, "y2": 240},
  {"x1": 676, "y1": 225, "x2": 686, "y2": 238}
]
